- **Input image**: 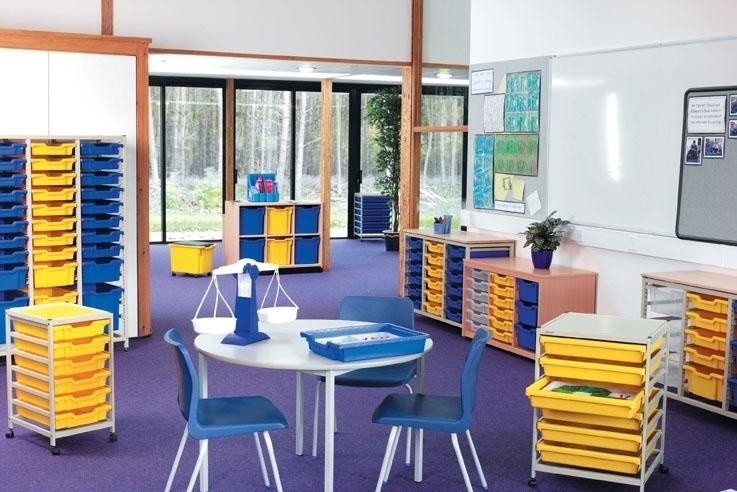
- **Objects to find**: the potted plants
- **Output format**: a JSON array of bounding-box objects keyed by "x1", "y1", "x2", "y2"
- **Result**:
[
  {"x1": 516, "y1": 210, "x2": 571, "y2": 268},
  {"x1": 362, "y1": 87, "x2": 430, "y2": 251}
]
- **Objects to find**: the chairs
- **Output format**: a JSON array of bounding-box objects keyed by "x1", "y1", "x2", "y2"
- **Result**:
[
  {"x1": 372, "y1": 327, "x2": 491, "y2": 492},
  {"x1": 162, "y1": 328, "x2": 289, "y2": 492},
  {"x1": 311, "y1": 295, "x2": 414, "y2": 466}
]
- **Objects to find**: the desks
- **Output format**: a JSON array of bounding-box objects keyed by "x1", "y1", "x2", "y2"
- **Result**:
[{"x1": 194, "y1": 320, "x2": 433, "y2": 492}]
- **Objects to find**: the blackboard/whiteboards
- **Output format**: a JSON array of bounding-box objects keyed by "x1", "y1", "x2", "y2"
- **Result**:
[{"x1": 676, "y1": 85, "x2": 737, "y2": 246}]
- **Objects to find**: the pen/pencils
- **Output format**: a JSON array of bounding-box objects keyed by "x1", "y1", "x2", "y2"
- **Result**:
[{"x1": 434, "y1": 216, "x2": 445, "y2": 224}]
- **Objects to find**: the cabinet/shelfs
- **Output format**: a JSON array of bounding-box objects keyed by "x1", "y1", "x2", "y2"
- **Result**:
[
  {"x1": 353, "y1": 193, "x2": 392, "y2": 241},
  {"x1": 462, "y1": 257, "x2": 596, "y2": 361},
  {"x1": 641, "y1": 264, "x2": 736, "y2": 421},
  {"x1": 525, "y1": 312, "x2": 669, "y2": 491},
  {"x1": 221, "y1": 200, "x2": 323, "y2": 271}
]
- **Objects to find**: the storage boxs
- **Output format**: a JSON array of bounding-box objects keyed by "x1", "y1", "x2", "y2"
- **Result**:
[
  {"x1": 5, "y1": 302, "x2": 117, "y2": 455},
  {"x1": 402, "y1": 228, "x2": 516, "y2": 328},
  {"x1": 525, "y1": 336, "x2": 661, "y2": 476},
  {"x1": 267, "y1": 238, "x2": 293, "y2": 265},
  {"x1": 295, "y1": 237, "x2": 320, "y2": 265},
  {"x1": 239, "y1": 238, "x2": 265, "y2": 264},
  {"x1": 239, "y1": 207, "x2": 265, "y2": 236},
  {"x1": 647, "y1": 283, "x2": 725, "y2": 404},
  {"x1": 170, "y1": 243, "x2": 216, "y2": 277},
  {"x1": 295, "y1": 207, "x2": 320, "y2": 234},
  {"x1": 267, "y1": 206, "x2": 293, "y2": 235},
  {"x1": 468, "y1": 267, "x2": 535, "y2": 348},
  {"x1": 0, "y1": 134, "x2": 130, "y2": 359}
]
